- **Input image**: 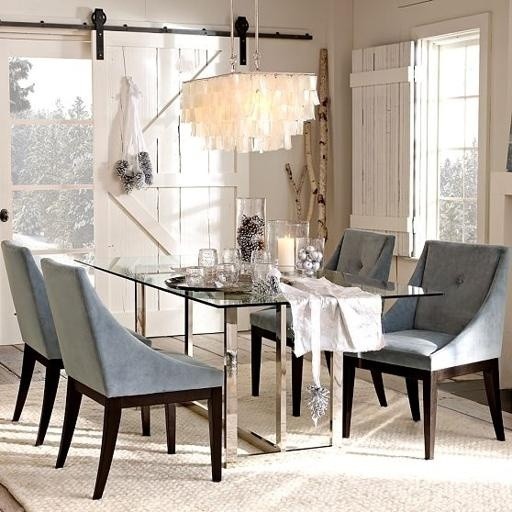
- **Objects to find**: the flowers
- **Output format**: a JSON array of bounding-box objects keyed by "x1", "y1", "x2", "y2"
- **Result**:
[{"x1": 237, "y1": 213, "x2": 265, "y2": 265}]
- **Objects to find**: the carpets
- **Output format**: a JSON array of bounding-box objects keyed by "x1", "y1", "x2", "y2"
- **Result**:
[{"x1": 0, "y1": 358, "x2": 511, "y2": 512}]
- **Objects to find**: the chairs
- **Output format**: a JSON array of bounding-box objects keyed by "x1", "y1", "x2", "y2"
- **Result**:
[
  {"x1": 343, "y1": 241, "x2": 512, "y2": 460},
  {"x1": 250, "y1": 227, "x2": 396, "y2": 418},
  {"x1": 40, "y1": 257, "x2": 223, "y2": 500},
  {"x1": 1, "y1": 240, "x2": 154, "y2": 448}
]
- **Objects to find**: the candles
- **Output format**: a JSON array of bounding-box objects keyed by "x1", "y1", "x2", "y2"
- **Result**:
[{"x1": 276, "y1": 236, "x2": 295, "y2": 273}]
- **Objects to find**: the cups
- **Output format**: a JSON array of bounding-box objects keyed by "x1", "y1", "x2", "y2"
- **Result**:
[
  {"x1": 197, "y1": 248, "x2": 219, "y2": 285},
  {"x1": 251, "y1": 249, "x2": 271, "y2": 282},
  {"x1": 220, "y1": 247, "x2": 242, "y2": 283},
  {"x1": 216, "y1": 262, "x2": 237, "y2": 288},
  {"x1": 185, "y1": 266, "x2": 207, "y2": 287}
]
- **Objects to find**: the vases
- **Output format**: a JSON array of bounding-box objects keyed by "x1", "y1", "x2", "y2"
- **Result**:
[
  {"x1": 235, "y1": 197, "x2": 266, "y2": 271},
  {"x1": 295, "y1": 238, "x2": 326, "y2": 276}
]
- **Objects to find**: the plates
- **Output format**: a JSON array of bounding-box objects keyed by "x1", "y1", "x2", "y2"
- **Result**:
[{"x1": 164, "y1": 274, "x2": 292, "y2": 292}]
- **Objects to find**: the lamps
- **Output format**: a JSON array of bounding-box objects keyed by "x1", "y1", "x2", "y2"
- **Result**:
[{"x1": 181, "y1": 0, "x2": 320, "y2": 156}]
